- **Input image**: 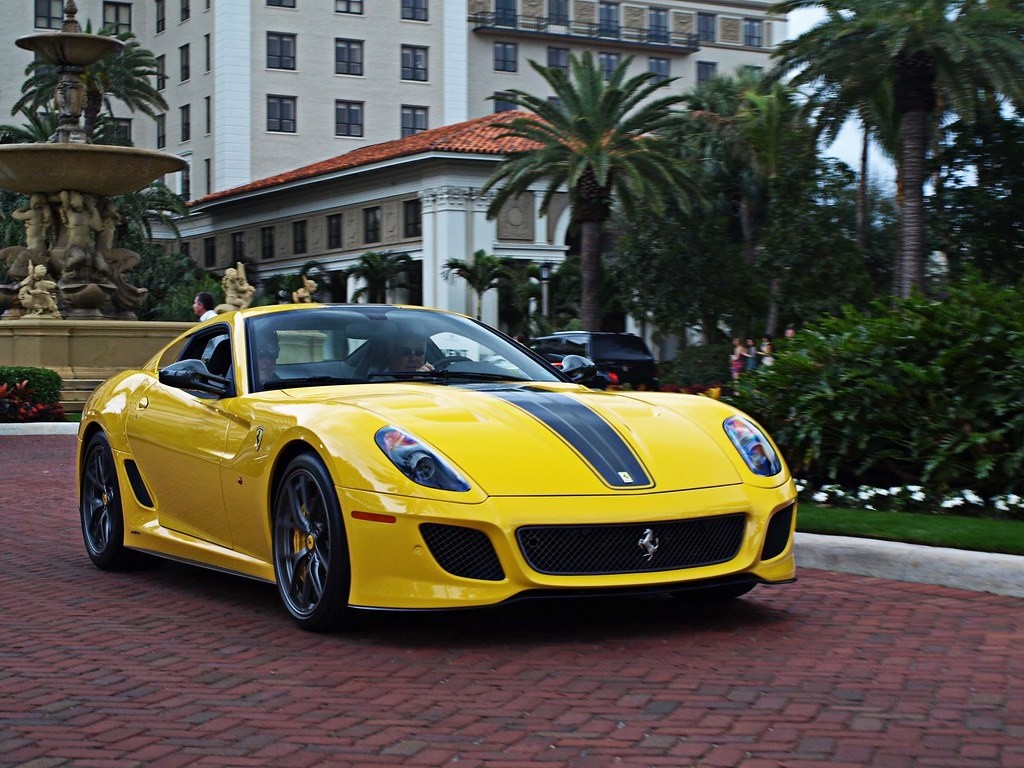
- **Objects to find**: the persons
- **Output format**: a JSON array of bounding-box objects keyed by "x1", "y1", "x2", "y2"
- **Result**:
[
  {"x1": 10, "y1": 192, "x2": 320, "y2": 319},
  {"x1": 758, "y1": 333, "x2": 777, "y2": 375},
  {"x1": 740, "y1": 338, "x2": 758, "y2": 371},
  {"x1": 226, "y1": 331, "x2": 281, "y2": 391},
  {"x1": 192, "y1": 293, "x2": 223, "y2": 323},
  {"x1": 784, "y1": 323, "x2": 797, "y2": 337},
  {"x1": 729, "y1": 336, "x2": 744, "y2": 398},
  {"x1": 366, "y1": 330, "x2": 437, "y2": 380}
]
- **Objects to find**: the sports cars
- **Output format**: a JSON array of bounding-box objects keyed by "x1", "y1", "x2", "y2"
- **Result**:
[{"x1": 75, "y1": 302, "x2": 798, "y2": 628}]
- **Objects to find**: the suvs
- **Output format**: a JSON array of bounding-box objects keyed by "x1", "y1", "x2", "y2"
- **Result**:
[{"x1": 525, "y1": 329, "x2": 655, "y2": 389}]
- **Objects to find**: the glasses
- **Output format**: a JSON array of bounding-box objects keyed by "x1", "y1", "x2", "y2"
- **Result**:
[
  {"x1": 192, "y1": 302, "x2": 198, "y2": 304},
  {"x1": 401, "y1": 350, "x2": 426, "y2": 357},
  {"x1": 258, "y1": 349, "x2": 277, "y2": 358}
]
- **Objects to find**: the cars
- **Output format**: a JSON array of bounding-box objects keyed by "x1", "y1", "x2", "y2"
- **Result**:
[{"x1": 483, "y1": 355, "x2": 519, "y2": 368}]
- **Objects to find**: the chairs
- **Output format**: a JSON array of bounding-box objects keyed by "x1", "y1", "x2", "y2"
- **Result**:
[
  {"x1": 207, "y1": 339, "x2": 232, "y2": 379},
  {"x1": 352, "y1": 340, "x2": 393, "y2": 380}
]
